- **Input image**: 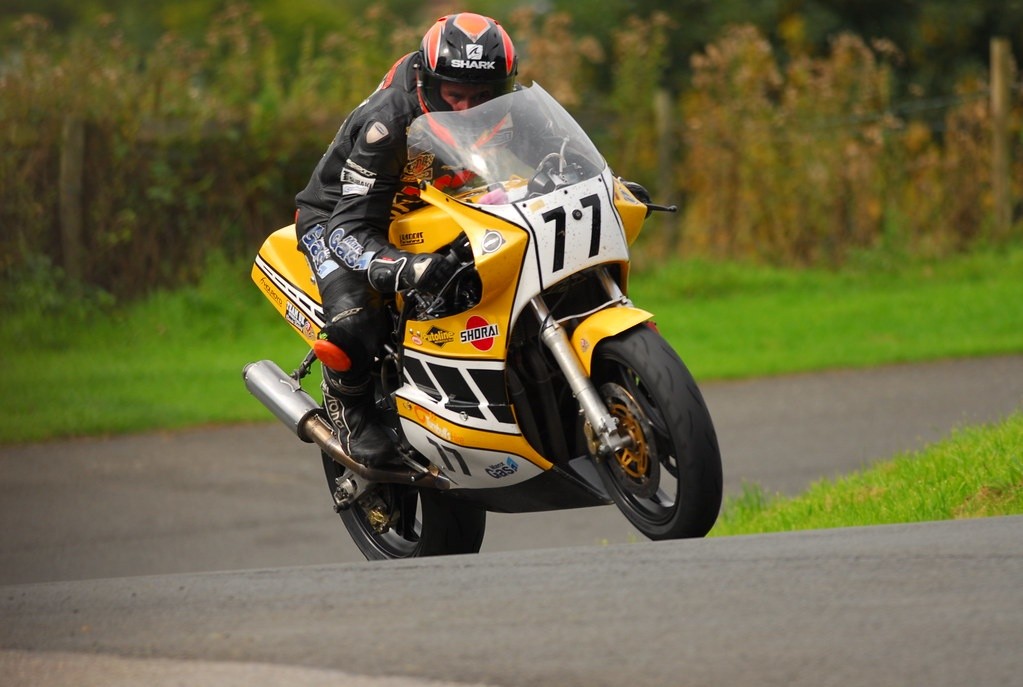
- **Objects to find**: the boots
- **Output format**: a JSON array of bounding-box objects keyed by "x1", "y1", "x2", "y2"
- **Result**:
[{"x1": 311, "y1": 292, "x2": 397, "y2": 466}]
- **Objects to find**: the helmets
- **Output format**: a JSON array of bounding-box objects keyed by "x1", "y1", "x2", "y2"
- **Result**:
[{"x1": 409, "y1": 10, "x2": 521, "y2": 156}]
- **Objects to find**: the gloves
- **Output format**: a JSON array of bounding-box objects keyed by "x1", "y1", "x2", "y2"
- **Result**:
[
  {"x1": 619, "y1": 178, "x2": 654, "y2": 220},
  {"x1": 367, "y1": 249, "x2": 458, "y2": 299}
]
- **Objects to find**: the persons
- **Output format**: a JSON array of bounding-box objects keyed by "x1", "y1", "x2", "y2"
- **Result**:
[{"x1": 294, "y1": 12, "x2": 652, "y2": 468}]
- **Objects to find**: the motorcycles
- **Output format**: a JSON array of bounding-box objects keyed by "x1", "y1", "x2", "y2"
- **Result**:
[{"x1": 240, "y1": 83, "x2": 724, "y2": 562}]
